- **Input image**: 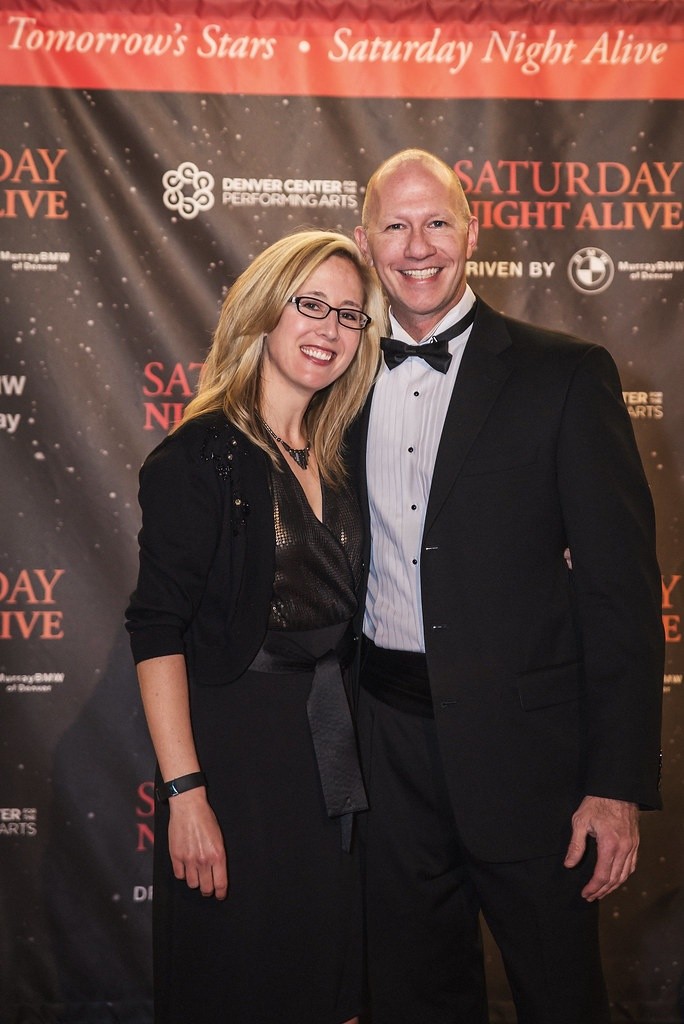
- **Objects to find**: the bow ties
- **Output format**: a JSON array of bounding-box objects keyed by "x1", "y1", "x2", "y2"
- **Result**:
[{"x1": 379, "y1": 301, "x2": 477, "y2": 375}]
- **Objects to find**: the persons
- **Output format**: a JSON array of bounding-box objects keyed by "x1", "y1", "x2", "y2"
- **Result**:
[{"x1": 125, "y1": 149, "x2": 664, "y2": 1023}]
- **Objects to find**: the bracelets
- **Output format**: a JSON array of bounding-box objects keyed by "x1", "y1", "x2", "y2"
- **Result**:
[{"x1": 156, "y1": 770, "x2": 206, "y2": 802}]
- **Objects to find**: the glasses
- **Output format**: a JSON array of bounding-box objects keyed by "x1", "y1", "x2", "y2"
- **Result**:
[{"x1": 287, "y1": 296, "x2": 371, "y2": 330}]
